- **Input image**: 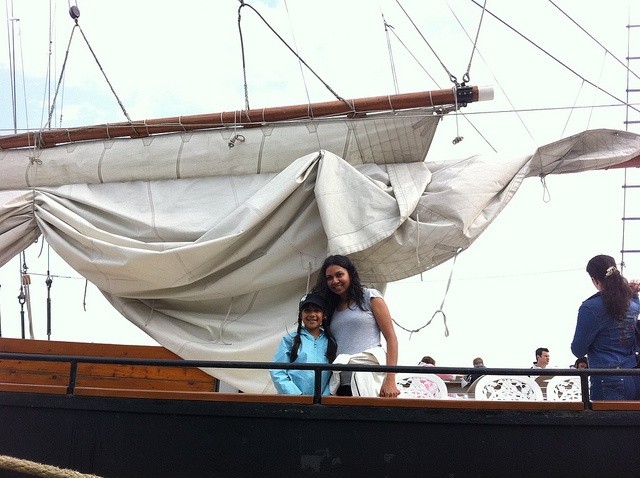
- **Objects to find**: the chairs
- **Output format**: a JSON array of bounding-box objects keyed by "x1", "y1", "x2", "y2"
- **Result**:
[
  {"x1": 393, "y1": 372, "x2": 448, "y2": 402},
  {"x1": 546, "y1": 374, "x2": 582, "y2": 403},
  {"x1": 474, "y1": 374, "x2": 544, "y2": 402}
]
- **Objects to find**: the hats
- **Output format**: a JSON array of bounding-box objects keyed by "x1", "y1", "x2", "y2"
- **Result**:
[{"x1": 298, "y1": 294, "x2": 327, "y2": 311}]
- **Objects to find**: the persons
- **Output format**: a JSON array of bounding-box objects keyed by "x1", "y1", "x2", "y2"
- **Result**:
[
  {"x1": 269, "y1": 293, "x2": 337, "y2": 396},
  {"x1": 464, "y1": 358, "x2": 487, "y2": 386},
  {"x1": 569, "y1": 357, "x2": 589, "y2": 369},
  {"x1": 571, "y1": 254, "x2": 640, "y2": 400},
  {"x1": 312, "y1": 255, "x2": 398, "y2": 398},
  {"x1": 417, "y1": 356, "x2": 453, "y2": 389},
  {"x1": 527, "y1": 348, "x2": 555, "y2": 382}
]
